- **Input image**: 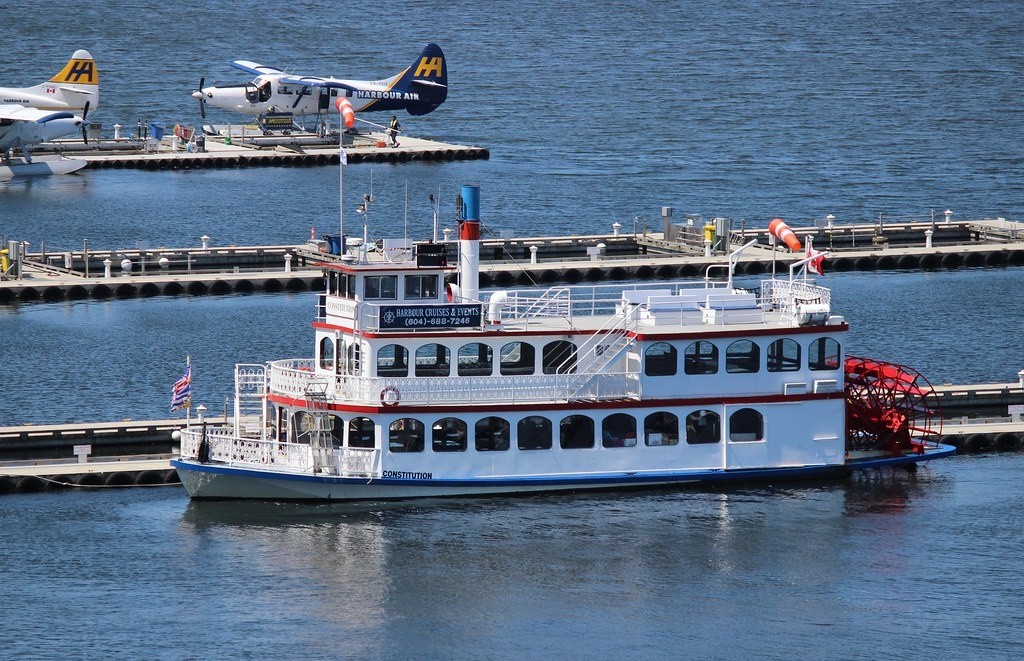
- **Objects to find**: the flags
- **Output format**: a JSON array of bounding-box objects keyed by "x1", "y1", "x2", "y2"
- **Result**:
[
  {"x1": 171, "y1": 364, "x2": 191, "y2": 412},
  {"x1": 807, "y1": 248, "x2": 826, "y2": 276}
]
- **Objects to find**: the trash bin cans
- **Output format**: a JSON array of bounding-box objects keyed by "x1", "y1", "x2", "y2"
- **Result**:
[
  {"x1": 322, "y1": 233, "x2": 347, "y2": 256},
  {"x1": 345, "y1": 237, "x2": 365, "y2": 263},
  {"x1": 150, "y1": 121, "x2": 166, "y2": 141}
]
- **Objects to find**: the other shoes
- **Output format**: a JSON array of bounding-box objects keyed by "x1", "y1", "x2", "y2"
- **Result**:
[{"x1": 389, "y1": 141, "x2": 400, "y2": 148}]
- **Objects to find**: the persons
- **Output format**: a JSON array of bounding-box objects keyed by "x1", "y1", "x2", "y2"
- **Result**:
[{"x1": 386, "y1": 116, "x2": 402, "y2": 148}]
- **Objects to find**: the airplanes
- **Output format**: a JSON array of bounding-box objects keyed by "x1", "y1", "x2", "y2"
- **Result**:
[
  {"x1": 0, "y1": 48, "x2": 99, "y2": 178},
  {"x1": 191, "y1": 41, "x2": 450, "y2": 145}
]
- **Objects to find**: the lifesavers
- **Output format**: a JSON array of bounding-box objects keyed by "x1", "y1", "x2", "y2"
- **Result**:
[{"x1": 380, "y1": 386, "x2": 401, "y2": 406}]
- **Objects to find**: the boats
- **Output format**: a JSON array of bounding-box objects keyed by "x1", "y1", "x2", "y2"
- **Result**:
[{"x1": 164, "y1": 98, "x2": 960, "y2": 510}]
